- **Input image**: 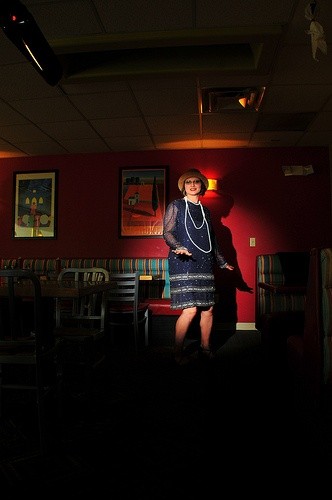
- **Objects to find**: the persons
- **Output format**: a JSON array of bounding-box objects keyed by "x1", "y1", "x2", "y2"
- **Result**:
[{"x1": 162, "y1": 168, "x2": 235, "y2": 368}]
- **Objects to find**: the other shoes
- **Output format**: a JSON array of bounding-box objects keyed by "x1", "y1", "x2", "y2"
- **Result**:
[{"x1": 198, "y1": 346, "x2": 217, "y2": 362}]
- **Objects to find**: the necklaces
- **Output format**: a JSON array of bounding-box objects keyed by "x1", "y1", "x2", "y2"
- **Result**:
[{"x1": 184, "y1": 196, "x2": 212, "y2": 254}]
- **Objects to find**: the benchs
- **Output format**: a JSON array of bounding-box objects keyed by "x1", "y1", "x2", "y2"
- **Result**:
[
  {"x1": 254, "y1": 250, "x2": 309, "y2": 337},
  {"x1": 0, "y1": 257, "x2": 185, "y2": 353}
]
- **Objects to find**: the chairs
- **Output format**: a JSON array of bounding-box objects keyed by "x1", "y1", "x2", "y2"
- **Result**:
[
  {"x1": 0, "y1": 270, "x2": 57, "y2": 448},
  {"x1": 109, "y1": 273, "x2": 149, "y2": 350},
  {"x1": 56, "y1": 266, "x2": 109, "y2": 381}
]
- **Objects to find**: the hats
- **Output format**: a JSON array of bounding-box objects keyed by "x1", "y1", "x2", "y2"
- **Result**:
[{"x1": 178, "y1": 168, "x2": 208, "y2": 192}]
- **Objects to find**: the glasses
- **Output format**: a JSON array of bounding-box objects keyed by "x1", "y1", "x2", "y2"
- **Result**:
[{"x1": 184, "y1": 180, "x2": 201, "y2": 184}]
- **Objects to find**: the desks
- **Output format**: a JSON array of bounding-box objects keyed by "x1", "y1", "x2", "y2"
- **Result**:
[
  {"x1": 0, "y1": 271, "x2": 164, "y2": 394},
  {"x1": 257, "y1": 281, "x2": 307, "y2": 295}
]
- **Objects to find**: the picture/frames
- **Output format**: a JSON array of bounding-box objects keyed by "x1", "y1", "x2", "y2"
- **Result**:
[
  {"x1": 116, "y1": 163, "x2": 171, "y2": 240},
  {"x1": 10, "y1": 169, "x2": 58, "y2": 241}
]
